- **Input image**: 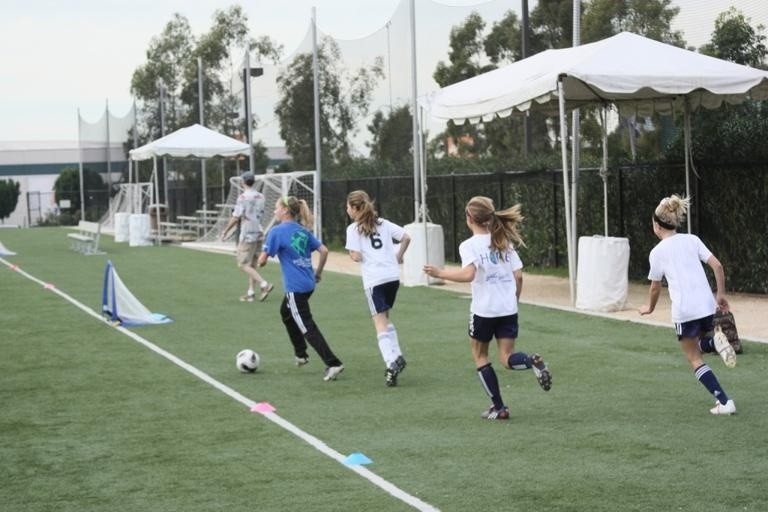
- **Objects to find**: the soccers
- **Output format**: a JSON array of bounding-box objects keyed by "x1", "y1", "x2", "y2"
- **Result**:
[{"x1": 237, "y1": 349, "x2": 260, "y2": 373}]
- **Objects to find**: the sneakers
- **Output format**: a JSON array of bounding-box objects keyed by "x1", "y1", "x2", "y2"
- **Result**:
[
  {"x1": 482, "y1": 405, "x2": 508, "y2": 420},
  {"x1": 324, "y1": 365, "x2": 343, "y2": 380},
  {"x1": 385, "y1": 356, "x2": 405, "y2": 386},
  {"x1": 714, "y1": 330, "x2": 737, "y2": 367},
  {"x1": 710, "y1": 399, "x2": 736, "y2": 415},
  {"x1": 240, "y1": 292, "x2": 254, "y2": 301},
  {"x1": 259, "y1": 283, "x2": 273, "y2": 300},
  {"x1": 530, "y1": 353, "x2": 552, "y2": 391},
  {"x1": 296, "y1": 356, "x2": 308, "y2": 364}
]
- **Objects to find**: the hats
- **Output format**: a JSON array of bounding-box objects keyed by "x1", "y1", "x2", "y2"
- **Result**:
[{"x1": 242, "y1": 171, "x2": 254, "y2": 184}]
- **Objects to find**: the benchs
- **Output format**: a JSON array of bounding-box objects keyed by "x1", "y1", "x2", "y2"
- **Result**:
[
  {"x1": 68, "y1": 221, "x2": 103, "y2": 255},
  {"x1": 153, "y1": 203, "x2": 241, "y2": 247}
]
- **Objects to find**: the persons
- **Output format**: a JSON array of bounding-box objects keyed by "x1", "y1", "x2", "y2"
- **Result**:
[
  {"x1": 636, "y1": 195, "x2": 738, "y2": 414},
  {"x1": 257, "y1": 195, "x2": 345, "y2": 381},
  {"x1": 346, "y1": 191, "x2": 412, "y2": 387},
  {"x1": 221, "y1": 171, "x2": 274, "y2": 301},
  {"x1": 422, "y1": 197, "x2": 552, "y2": 420}
]
